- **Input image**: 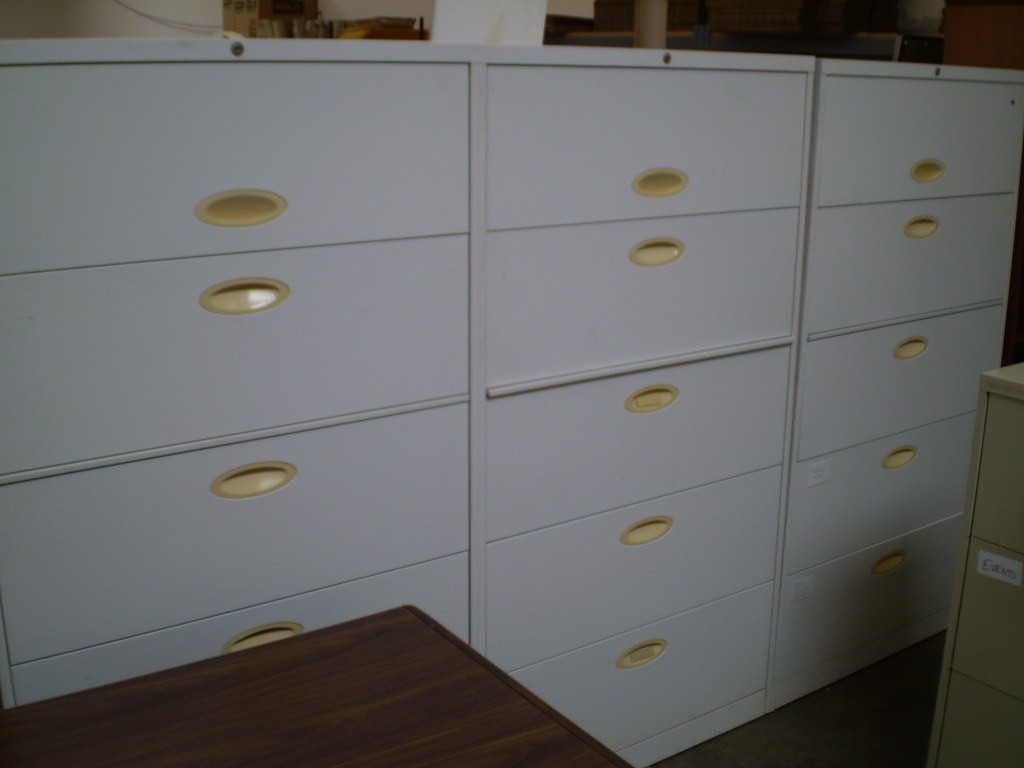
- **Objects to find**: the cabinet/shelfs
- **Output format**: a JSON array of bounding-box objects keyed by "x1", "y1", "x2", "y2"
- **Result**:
[
  {"x1": 928, "y1": 363, "x2": 1024, "y2": 768},
  {"x1": 762, "y1": 57, "x2": 1024, "y2": 713},
  {"x1": 0, "y1": 34, "x2": 818, "y2": 768}
]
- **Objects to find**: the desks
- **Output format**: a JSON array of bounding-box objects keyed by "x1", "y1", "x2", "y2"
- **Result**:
[{"x1": 0, "y1": 604, "x2": 634, "y2": 768}]
